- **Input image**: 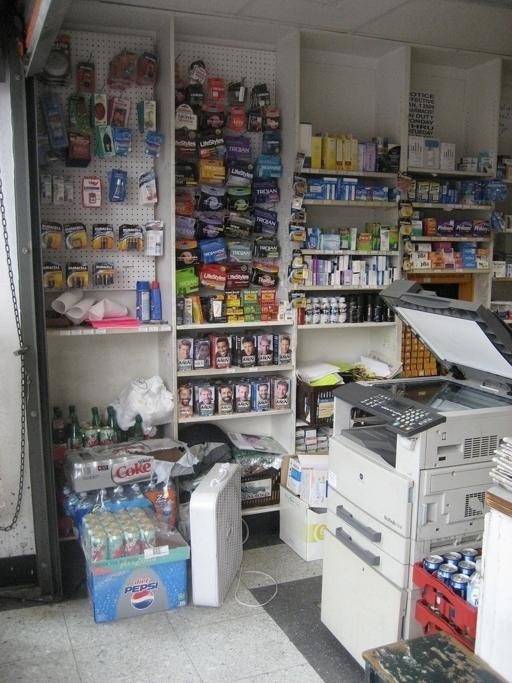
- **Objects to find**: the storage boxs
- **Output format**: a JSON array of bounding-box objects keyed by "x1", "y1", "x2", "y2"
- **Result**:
[
  {"x1": 274, "y1": 451, "x2": 328, "y2": 561},
  {"x1": 85, "y1": 554, "x2": 187, "y2": 624}
]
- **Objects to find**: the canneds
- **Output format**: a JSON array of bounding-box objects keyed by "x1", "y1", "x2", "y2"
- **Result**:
[
  {"x1": 82, "y1": 507, "x2": 157, "y2": 561},
  {"x1": 79, "y1": 422, "x2": 114, "y2": 447},
  {"x1": 424, "y1": 548, "x2": 478, "y2": 617}
]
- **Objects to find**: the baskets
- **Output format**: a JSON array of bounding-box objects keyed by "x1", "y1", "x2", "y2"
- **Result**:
[
  {"x1": 415, "y1": 599, "x2": 474, "y2": 653},
  {"x1": 413, "y1": 547, "x2": 482, "y2": 639},
  {"x1": 297, "y1": 375, "x2": 356, "y2": 427},
  {"x1": 241, "y1": 467, "x2": 280, "y2": 510}
]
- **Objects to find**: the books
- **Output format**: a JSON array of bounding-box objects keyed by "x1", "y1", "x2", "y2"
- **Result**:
[{"x1": 489, "y1": 437, "x2": 512, "y2": 492}]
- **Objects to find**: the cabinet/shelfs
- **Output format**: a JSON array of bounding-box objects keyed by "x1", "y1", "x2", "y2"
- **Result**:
[{"x1": 26, "y1": 2, "x2": 512, "y2": 540}]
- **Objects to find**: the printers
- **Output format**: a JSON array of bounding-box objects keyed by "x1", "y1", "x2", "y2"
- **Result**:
[{"x1": 320, "y1": 279, "x2": 512, "y2": 672}]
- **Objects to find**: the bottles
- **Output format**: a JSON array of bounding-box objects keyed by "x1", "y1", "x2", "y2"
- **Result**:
[
  {"x1": 150, "y1": 281, "x2": 162, "y2": 320},
  {"x1": 296, "y1": 293, "x2": 397, "y2": 325},
  {"x1": 51, "y1": 404, "x2": 142, "y2": 465},
  {"x1": 62, "y1": 480, "x2": 177, "y2": 533}
]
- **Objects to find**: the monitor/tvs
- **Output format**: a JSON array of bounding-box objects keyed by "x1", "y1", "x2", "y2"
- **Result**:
[{"x1": 25, "y1": 0, "x2": 73, "y2": 78}]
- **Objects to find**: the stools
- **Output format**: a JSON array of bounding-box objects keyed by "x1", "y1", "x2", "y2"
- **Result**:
[{"x1": 358, "y1": 629, "x2": 510, "y2": 683}]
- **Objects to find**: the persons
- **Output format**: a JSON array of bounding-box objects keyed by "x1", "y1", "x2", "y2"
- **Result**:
[
  {"x1": 199, "y1": 389, "x2": 214, "y2": 414},
  {"x1": 197, "y1": 342, "x2": 210, "y2": 368},
  {"x1": 242, "y1": 337, "x2": 256, "y2": 366},
  {"x1": 258, "y1": 335, "x2": 272, "y2": 364},
  {"x1": 215, "y1": 338, "x2": 231, "y2": 366},
  {"x1": 177, "y1": 387, "x2": 192, "y2": 417},
  {"x1": 275, "y1": 381, "x2": 289, "y2": 409},
  {"x1": 280, "y1": 337, "x2": 292, "y2": 364},
  {"x1": 238, "y1": 384, "x2": 250, "y2": 411},
  {"x1": 255, "y1": 383, "x2": 268, "y2": 410},
  {"x1": 179, "y1": 340, "x2": 191, "y2": 369},
  {"x1": 219, "y1": 386, "x2": 233, "y2": 415}
]
